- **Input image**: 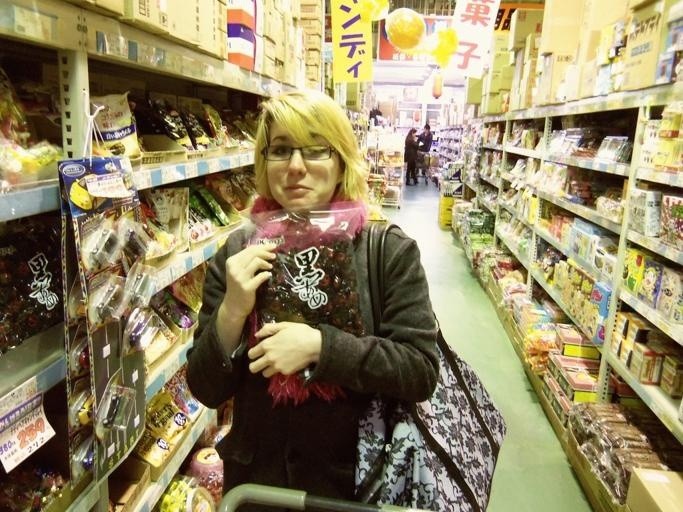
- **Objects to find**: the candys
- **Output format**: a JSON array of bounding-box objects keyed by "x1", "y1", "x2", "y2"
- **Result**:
[{"x1": 155, "y1": 470, "x2": 224, "y2": 512}]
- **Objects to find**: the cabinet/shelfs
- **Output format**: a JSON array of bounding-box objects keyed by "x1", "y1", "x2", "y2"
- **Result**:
[
  {"x1": 0, "y1": 1, "x2": 269, "y2": 512},
  {"x1": 458, "y1": 82, "x2": 683, "y2": 444}
]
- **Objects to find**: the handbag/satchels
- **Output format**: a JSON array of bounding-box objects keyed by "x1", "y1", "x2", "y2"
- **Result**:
[{"x1": 354, "y1": 311, "x2": 507, "y2": 511}]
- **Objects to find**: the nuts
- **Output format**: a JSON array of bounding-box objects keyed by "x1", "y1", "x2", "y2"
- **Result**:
[
  {"x1": 257, "y1": 236, "x2": 365, "y2": 371},
  {"x1": 0, "y1": 211, "x2": 67, "y2": 351}
]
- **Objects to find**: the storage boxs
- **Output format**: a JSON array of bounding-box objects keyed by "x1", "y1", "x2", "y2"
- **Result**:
[
  {"x1": 94, "y1": 1, "x2": 322, "y2": 90},
  {"x1": 480, "y1": 0, "x2": 660, "y2": 117}
]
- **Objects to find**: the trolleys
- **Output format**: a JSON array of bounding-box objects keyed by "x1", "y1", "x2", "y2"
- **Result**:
[{"x1": 415, "y1": 147, "x2": 440, "y2": 187}]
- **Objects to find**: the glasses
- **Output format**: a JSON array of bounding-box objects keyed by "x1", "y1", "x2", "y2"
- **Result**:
[{"x1": 261, "y1": 143, "x2": 336, "y2": 161}]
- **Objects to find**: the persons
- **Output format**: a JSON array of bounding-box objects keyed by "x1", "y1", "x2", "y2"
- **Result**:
[
  {"x1": 414, "y1": 125, "x2": 433, "y2": 178},
  {"x1": 184, "y1": 88, "x2": 440, "y2": 511},
  {"x1": 403, "y1": 127, "x2": 422, "y2": 186}
]
